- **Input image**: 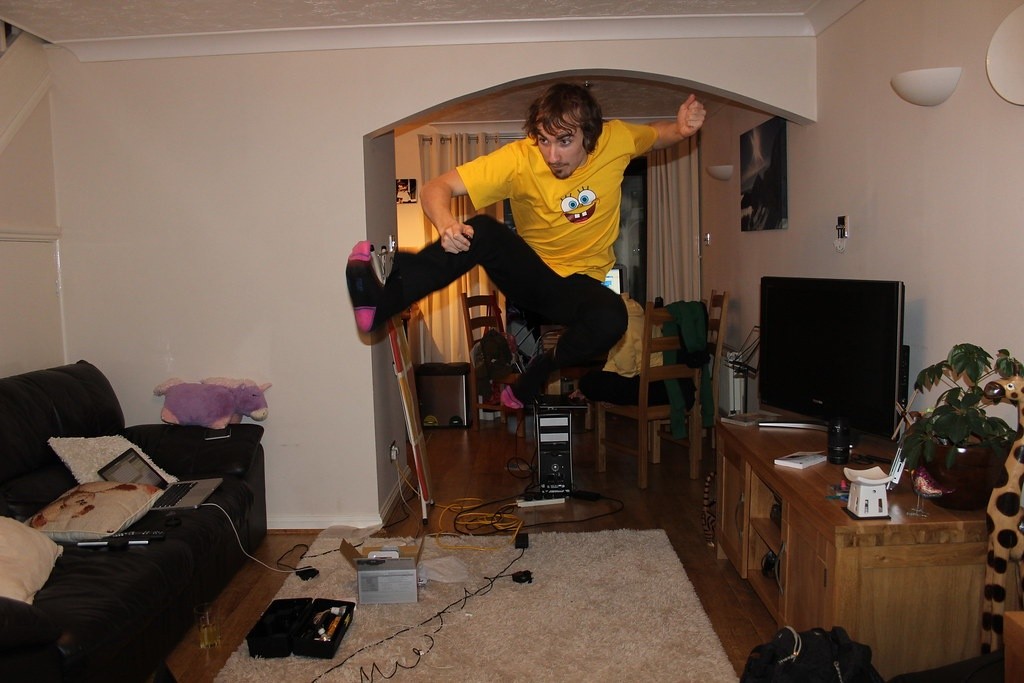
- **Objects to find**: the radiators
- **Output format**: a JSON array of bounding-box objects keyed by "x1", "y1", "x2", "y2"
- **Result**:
[{"x1": 708, "y1": 343, "x2": 745, "y2": 417}]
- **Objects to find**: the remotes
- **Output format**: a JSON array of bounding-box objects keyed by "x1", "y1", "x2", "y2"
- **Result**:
[{"x1": 75, "y1": 530, "x2": 167, "y2": 546}]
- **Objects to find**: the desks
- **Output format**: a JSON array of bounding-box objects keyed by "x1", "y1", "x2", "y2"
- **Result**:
[{"x1": 541, "y1": 327, "x2": 594, "y2": 430}]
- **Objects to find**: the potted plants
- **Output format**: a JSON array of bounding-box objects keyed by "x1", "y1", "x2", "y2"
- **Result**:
[{"x1": 898, "y1": 343, "x2": 1024, "y2": 511}]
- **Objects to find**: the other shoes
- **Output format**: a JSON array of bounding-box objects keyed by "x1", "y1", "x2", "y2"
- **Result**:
[
  {"x1": 424, "y1": 415, "x2": 439, "y2": 425},
  {"x1": 449, "y1": 415, "x2": 463, "y2": 425}
]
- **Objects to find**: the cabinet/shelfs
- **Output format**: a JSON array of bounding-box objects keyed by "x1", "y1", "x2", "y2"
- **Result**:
[{"x1": 717, "y1": 413, "x2": 988, "y2": 683}]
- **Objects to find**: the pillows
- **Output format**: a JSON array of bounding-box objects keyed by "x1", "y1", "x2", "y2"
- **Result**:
[
  {"x1": 23, "y1": 481, "x2": 164, "y2": 542},
  {"x1": 0, "y1": 516, "x2": 64, "y2": 605},
  {"x1": 47, "y1": 434, "x2": 180, "y2": 491}
]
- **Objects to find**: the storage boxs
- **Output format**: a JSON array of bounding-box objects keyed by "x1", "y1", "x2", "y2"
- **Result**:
[{"x1": 339, "y1": 532, "x2": 426, "y2": 605}]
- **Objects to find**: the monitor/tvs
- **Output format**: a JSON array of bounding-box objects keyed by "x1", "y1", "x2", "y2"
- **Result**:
[
  {"x1": 757, "y1": 275, "x2": 910, "y2": 449},
  {"x1": 600, "y1": 264, "x2": 627, "y2": 295}
]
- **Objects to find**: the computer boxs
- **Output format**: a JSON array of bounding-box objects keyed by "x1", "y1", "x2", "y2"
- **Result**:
[{"x1": 534, "y1": 411, "x2": 575, "y2": 494}]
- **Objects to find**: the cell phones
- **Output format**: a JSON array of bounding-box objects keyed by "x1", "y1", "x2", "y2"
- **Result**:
[{"x1": 204, "y1": 427, "x2": 231, "y2": 441}]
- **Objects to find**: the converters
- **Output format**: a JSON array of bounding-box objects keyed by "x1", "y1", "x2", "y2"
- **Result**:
[
  {"x1": 573, "y1": 490, "x2": 601, "y2": 502},
  {"x1": 515, "y1": 532, "x2": 529, "y2": 549},
  {"x1": 297, "y1": 566, "x2": 319, "y2": 581}
]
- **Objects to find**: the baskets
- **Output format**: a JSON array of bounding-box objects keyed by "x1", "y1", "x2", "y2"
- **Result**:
[{"x1": 702, "y1": 471, "x2": 717, "y2": 546}]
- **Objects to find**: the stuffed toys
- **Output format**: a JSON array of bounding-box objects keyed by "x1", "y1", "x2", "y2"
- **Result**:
[{"x1": 153, "y1": 377, "x2": 272, "y2": 429}]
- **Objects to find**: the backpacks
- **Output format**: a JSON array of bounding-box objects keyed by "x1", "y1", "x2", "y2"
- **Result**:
[{"x1": 739, "y1": 625, "x2": 883, "y2": 683}]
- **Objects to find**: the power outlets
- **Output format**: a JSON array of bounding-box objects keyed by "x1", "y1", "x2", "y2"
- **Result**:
[{"x1": 389, "y1": 440, "x2": 396, "y2": 464}]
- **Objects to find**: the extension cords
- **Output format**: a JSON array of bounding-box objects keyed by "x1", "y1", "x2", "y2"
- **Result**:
[{"x1": 515, "y1": 496, "x2": 567, "y2": 507}]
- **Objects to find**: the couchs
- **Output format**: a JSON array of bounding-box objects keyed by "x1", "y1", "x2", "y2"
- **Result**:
[{"x1": 0, "y1": 359, "x2": 268, "y2": 683}]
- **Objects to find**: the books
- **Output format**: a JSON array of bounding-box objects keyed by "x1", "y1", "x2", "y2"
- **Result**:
[
  {"x1": 774, "y1": 451, "x2": 827, "y2": 469},
  {"x1": 721, "y1": 413, "x2": 777, "y2": 426}
]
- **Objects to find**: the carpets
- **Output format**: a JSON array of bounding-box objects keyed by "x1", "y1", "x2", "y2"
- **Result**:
[{"x1": 212, "y1": 529, "x2": 740, "y2": 683}]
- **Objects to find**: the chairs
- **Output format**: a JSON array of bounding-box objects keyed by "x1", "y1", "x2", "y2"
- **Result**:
[
  {"x1": 598, "y1": 298, "x2": 707, "y2": 489},
  {"x1": 647, "y1": 289, "x2": 730, "y2": 464},
  {"x1": 461, "y1": 290, "x2": 549, "y2": 437}
]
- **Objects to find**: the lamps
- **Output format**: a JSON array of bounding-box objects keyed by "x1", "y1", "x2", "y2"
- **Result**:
[
  {"x1": 706, "y1": 164, "x2": 734, "y2": 181},
  {"x1": 888, "y1": 66, "x2": 962, "y2": 108}
]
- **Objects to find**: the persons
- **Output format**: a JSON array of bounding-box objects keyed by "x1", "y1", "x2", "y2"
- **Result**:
[
  {"x1": 569, "y1": 293, "x2": 663, "y2": 405},
  {"x1": 346, "y1": 82, "x2": 706, "y2": 410}
]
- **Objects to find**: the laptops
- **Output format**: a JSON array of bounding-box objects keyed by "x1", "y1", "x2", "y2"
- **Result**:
[
  {"x1": 96, "y1": 447, "x2": 226, "y2": 511},
  {"x1": 513, "y1": 360, "x2": 591, "y2": 409}
]
